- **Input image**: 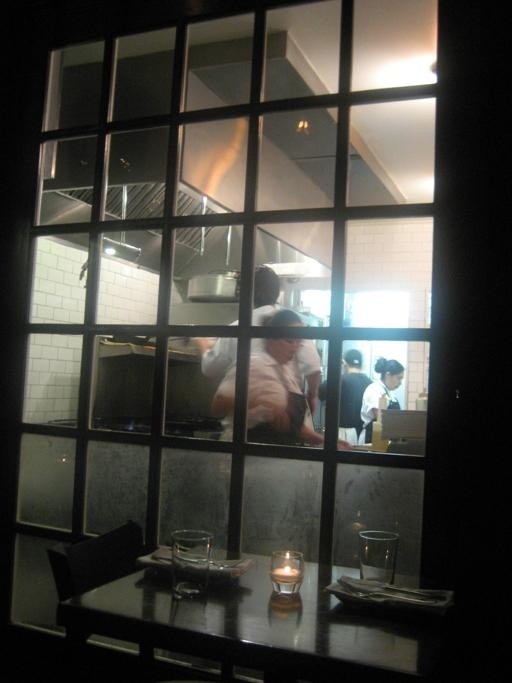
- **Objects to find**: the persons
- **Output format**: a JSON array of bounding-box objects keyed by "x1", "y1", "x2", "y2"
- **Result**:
[
  {"x1": 356, "y1": 354, "x2": 405, "y2": 445},
  {"x1": 214, "y1": 305, "x2": 350, "y2": 535},
  {"x1": 187, "y1": 263, "x2": 323, "y2": 439},
  {"x1": 318, "y1": 346, "x2": 376, "y2": 444}
]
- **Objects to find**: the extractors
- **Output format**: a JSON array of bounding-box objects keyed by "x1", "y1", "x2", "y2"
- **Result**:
[{"x1": 40, "y1": 35, "x2": 353, "y2": 304}]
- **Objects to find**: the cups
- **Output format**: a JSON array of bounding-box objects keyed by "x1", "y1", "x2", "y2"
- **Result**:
[
  {"x1": 357, "y1": 531, "x2": 400, "y2": 586},
  {"x1": 270, "y1": 551, "x2": 305, "y2": 596}
]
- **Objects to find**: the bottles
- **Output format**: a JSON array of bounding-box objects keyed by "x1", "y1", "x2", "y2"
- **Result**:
[
  {"x1": 371, "y1": 410, "x2": 389, "y2": 451},
  {"x1": 169, "y1": 529, "x2": 212, "y2": 601},
  {"x1": 416, "y1": 389, "x2": 427, "y2": 411}
]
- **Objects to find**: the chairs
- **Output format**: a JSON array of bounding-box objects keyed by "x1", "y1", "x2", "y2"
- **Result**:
[{"x1": 45, "y1": 519, "x2": 151, "y2": 600}]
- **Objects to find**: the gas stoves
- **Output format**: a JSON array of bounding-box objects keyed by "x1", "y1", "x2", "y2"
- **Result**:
[{"x1": 48, "y1": 415, "x2": 225, "y2": 443}]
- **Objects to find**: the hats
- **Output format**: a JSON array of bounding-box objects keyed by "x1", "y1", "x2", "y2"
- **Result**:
[{"x1": 342, "y1": 347, "x2": 362, "y2": 364}]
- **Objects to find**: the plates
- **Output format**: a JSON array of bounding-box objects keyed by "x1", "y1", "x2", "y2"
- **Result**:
[{"x1": 351, "y1": 443, "x2": 371, "y2": 451}]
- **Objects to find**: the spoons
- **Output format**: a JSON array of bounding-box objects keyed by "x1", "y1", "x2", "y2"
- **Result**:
[
  {"x1": 150, "y1": 554, "x2": 246, "y2": 568},
  {"x1": 337, "y1": 579, "x2": 435, "y2": 607}
]
